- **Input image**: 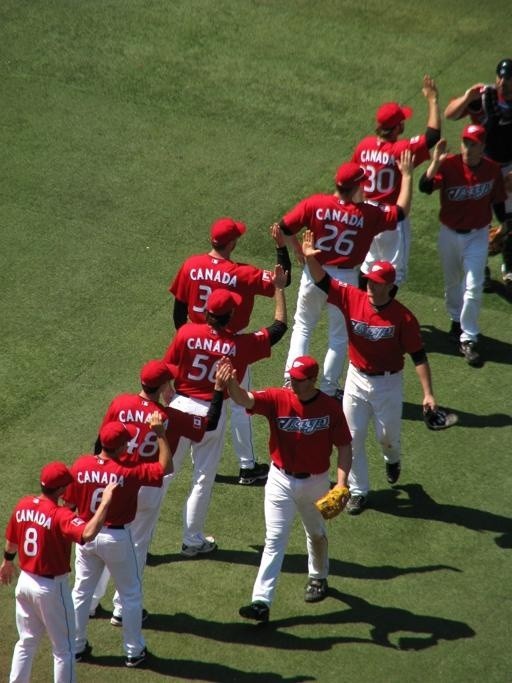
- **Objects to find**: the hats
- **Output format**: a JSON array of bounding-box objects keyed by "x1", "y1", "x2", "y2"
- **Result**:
[
  {"x1": 205, "y1": 288, "x2": 243, "y2": 316},
  {"x1": 375, "y1": 103, "x2": 413, "y2": 129},
  {"x1": 361, "y1": 260, "x2": 395, "y2": 284},
  {"x1": 40, "y1": 360, "x2": 179, "y2": 488},
  {"x1": 284, "y1": 356, "x2": 318, "y2": 380},
  {"x1": 335, "y1": 161, "x2": 369, "y2": 188},
  {"x1": 462, "y1": 125, "x2": 487, "y2": 145},
  {"x1": 211, "y1": 218, "x2": 246, "y2": 245},
  {"x1": 498, "y1": 59, "x2": 511, "y2": 76}
]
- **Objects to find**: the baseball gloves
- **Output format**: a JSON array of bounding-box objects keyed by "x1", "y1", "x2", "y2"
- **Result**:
[
  {"x1": 504, "y1": 171, "x2": 512, "y2": 192},
  {"x1": 488, "y1": 227, "x2": 512, "y2": 256},
  {"x1": 423, "y1": 406, "x2": 459, "y2": 430},
  {"x1": 314, "y1": 484, "x2": 351, "y2": 519}
]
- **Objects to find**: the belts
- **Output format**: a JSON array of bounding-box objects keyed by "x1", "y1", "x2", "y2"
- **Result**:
[{"x1": 273, "y1": 464, "x2": 311, "y2": 479}]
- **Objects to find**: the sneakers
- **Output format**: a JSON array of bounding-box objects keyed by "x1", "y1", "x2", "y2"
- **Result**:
[
  {"x1": 385, "y1": 461, "x2": 401, "y2": 483},
  {"x1": 237, "y1": 462, "x2": 269, "y2": 485},
  {"x1": 346, "y1": 496, "x2": 368, "y2": 514},
  {"x1": 239, "y1": 600, "x2": 269, "y2": 621},
  {"x1": 180, "y1": 536, "x2": 215, "y2": 558},
  {"x1": 451, "y1": 321, "x2": 481, "y2": 366},
  {"x1": 76, "y1": 602, "x2": 149, "y2": 667},
  {"x1": 304, "y1": 578, "x2": 328, "y2": 602}
]
